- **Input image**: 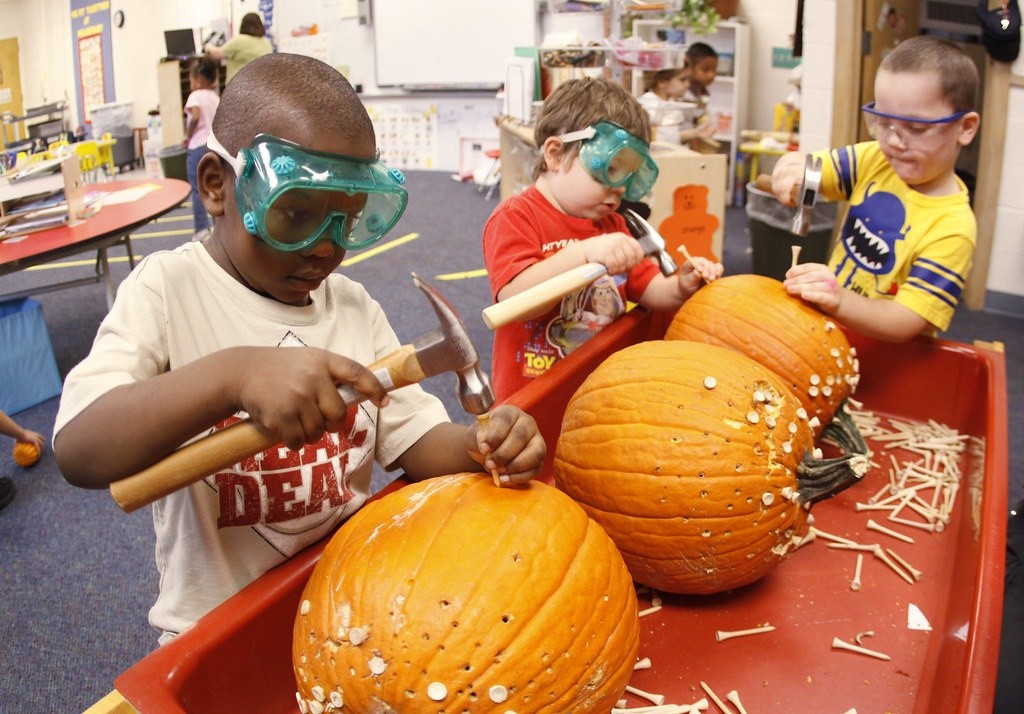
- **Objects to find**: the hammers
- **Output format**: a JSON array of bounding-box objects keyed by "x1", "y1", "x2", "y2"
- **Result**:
[
  {"x1": 481, "y1": 208, "x2": 679, "y2": 331},
  {"x1": 756, "y1": 153, "x2": 823, "y2": 238},
  {"x1": 109, "y1": 272, "x2": 496, "y2": 512}
]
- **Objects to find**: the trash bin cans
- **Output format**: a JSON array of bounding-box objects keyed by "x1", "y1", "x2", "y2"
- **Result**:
[
  {"x1": 744, "y1": 179, "x2": 839, "y2": 283},
  {"x1": 88, "y1": 99, "x2": 135, "y2": 176}
]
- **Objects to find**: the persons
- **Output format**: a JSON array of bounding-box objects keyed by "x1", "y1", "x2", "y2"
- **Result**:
[
  {"x1": 0, "y1": 410, "x2": 46, "y2": 512},
  {"x1": 180, "y1": 56, "x2": 221, "y2": 245},
  {"x1": 206, "y1": 12, "x2": 273, "y2": 87},
  {"x1": 51, "y1": 54, "x2": 548, "y2": 647},
  {"x1": 482, "y1": 74, "x2": 725, "y2": 411},
  {"x1": 638, "y1": 40, "x2": 719, "y2": 154},
  {"x1": 774, "y1": 34, "x2": 978, "y2": 344}
]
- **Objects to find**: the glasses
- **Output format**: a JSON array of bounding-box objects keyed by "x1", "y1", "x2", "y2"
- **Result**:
[
  {"x1": 580, "y1": 120, "x2": 659, "y2": 202},
  {"x1": 862, "y1": 102, "x2": 965, "y2": 149},
  {"x1": 235, "y1": 133, "x2": 408, "y2": 252}
]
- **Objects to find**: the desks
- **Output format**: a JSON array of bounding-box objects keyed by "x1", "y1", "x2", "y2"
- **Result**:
[{"x1": 0, "y1": 177, "x2": 192, "y2": 314}]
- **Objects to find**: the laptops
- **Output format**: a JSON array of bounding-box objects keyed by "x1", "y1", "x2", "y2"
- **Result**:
[
  {"x1": 165, "y1": 29, "x2": 195, "y2": 57},
  {"x1": 1, "y1": 154, "x2": 82, "y2": 239}
]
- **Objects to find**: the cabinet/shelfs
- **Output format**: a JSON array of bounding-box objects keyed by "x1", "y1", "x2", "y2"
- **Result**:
[
  {"x1": 538, "y1": 19, "x2": 751, "y2": 206},
  {"x1": 496, "y1": 118, "x2": 728, "y2": 277}
]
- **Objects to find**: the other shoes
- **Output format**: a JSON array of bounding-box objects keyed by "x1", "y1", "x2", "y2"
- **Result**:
[
  {"x1": 192, "y1": 228, "x2": 212, "y2": 243},
  {"x1": 0, "y1": 478, "x2": 16, "y2": 509}
]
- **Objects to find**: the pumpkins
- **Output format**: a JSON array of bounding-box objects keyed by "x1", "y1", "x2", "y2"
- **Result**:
[
  {"x1": 552, "y1": 340, "x2": 869, "y2": 595},
  {"x1": 663, "y1": 273, "x2": 870, "y2": 459},
  {"x1": 291, "y1": 471, "x2": 640, "y2": 714},
  {"x1": 13, "y1": 442, "x2": 39, "y2": 467}
]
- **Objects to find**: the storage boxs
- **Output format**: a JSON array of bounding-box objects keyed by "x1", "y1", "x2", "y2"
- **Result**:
[{"x1": 0, "y1": 297, "x2": 63, "y2": 416}]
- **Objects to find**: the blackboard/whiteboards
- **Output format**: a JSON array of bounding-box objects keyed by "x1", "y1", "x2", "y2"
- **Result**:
[{"x1": 369, "y1": 0, "x2": 536, "y2": 92}]
